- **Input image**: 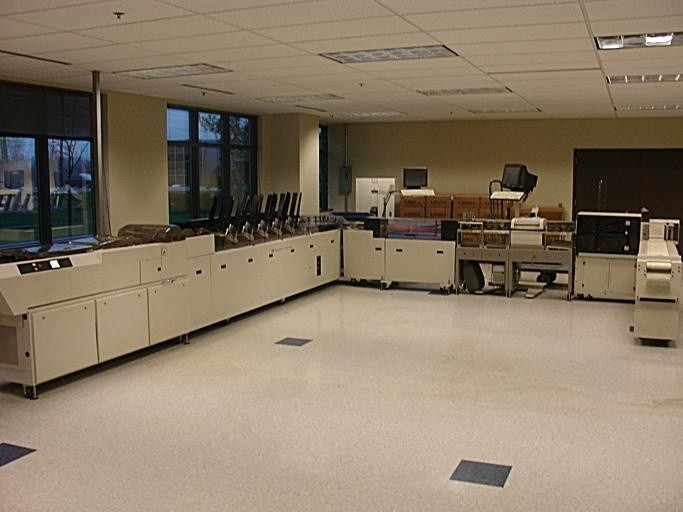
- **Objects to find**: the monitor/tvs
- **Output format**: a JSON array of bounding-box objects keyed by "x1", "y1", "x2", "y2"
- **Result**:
[
  {"x1": 501, "y1": 164, "x2": 538, "y2": 194},
  {"x1": 403, "y1": 167, "x2": 429, "y2": 189}
]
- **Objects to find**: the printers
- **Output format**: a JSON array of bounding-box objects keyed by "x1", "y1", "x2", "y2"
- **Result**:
[{"x1": 511, "y1": 217, "x2": 548, "y2": 245}]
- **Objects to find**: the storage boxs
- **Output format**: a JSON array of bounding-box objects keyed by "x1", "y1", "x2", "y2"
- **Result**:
[{"x1": 400, "y1": 195, "x2": 565, "y2": 244}]
